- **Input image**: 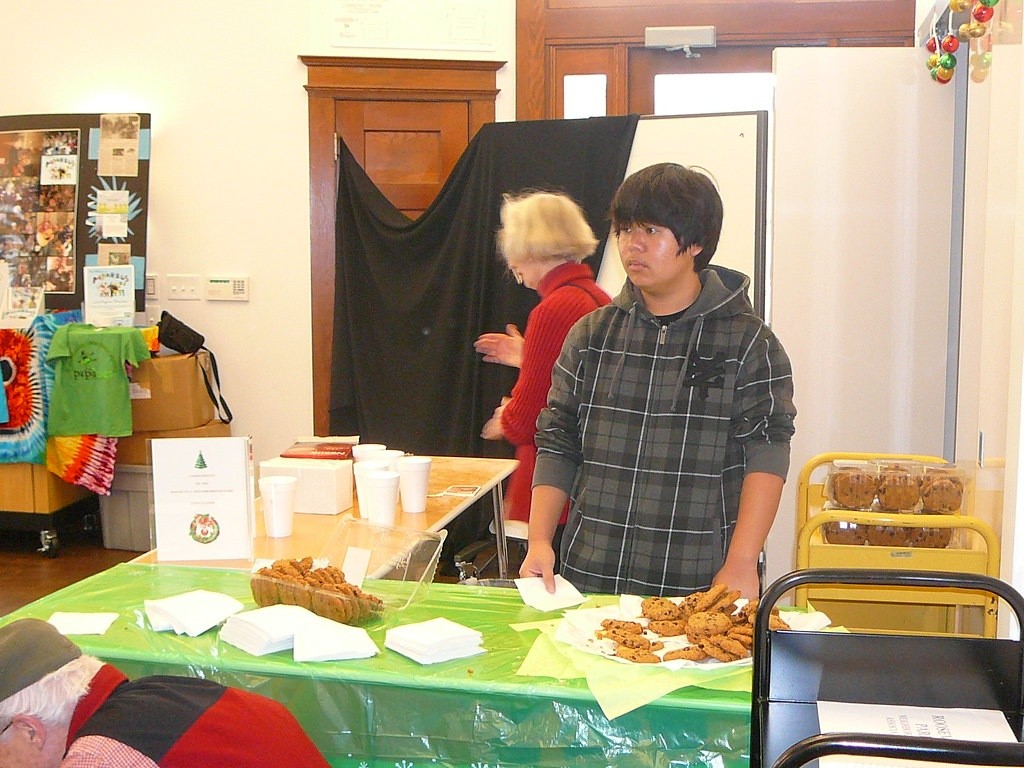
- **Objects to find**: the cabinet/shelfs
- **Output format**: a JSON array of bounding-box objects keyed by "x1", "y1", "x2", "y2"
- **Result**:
[{"x1": 0, "y1": 463, "x2": 103, "y2": 559}]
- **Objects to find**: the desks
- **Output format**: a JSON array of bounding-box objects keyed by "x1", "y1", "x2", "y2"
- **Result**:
[{"x1": 128, "y1": 456, "x2": 521, "y2": 580}]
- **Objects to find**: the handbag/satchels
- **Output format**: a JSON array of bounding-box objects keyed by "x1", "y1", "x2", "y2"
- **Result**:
[{"x1": 158, "y1": 311, "x2": 205, "y2": 354}]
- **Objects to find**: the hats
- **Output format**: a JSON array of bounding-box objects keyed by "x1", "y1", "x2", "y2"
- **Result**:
[{"x1": 0, "y1": 618, "x2": 83, "y2": 703}]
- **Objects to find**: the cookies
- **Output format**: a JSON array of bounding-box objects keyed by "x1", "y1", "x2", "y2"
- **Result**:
[
  {"x1": 822, "y1": 463, "x2": 963, "y2": 550},
  {"x1": 247, "y1": 556, "x2": 387, "y2": 624},
  {"x1": 593, "y1": 583, "x2": 791, "y2": 664}
]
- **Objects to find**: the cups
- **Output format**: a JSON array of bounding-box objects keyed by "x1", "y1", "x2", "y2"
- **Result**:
[
  {"x1": 365, "y1": 471, "x2": 399, "y2": 526},
  {"x1": 354, "y1": 462, "x2": 389, "y2": 518},
  {"x1": 352, "y1": 444, "x2": 387, "y2": 463},
  {"x1": 397, "y1": 456, "x2": 432, "y2": 512},
  {"x1": 371, "y1": 449, "x2": 405, "y2": 503},
  {"x1": 258, "y1": 475, "x2": 300, "y2": 537}
]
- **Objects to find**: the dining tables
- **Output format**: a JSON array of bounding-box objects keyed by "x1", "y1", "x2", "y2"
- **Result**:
[{"x1": 0, "y1": 562, "x2": 809, "y2": 768}]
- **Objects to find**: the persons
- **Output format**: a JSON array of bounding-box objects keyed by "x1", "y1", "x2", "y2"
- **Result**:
[
  {"x1": 0, "y1": 618, "x2": 331, "y2": 768},
  {"x1": 520, "y1": 163, "x2": 796, "y2": 604},
  {"x1": 0, "y1": 130, "x2": 79, "y2": 291},
  {"x1": 474, "y1": 192, "x2": 615, "y2": 575}
]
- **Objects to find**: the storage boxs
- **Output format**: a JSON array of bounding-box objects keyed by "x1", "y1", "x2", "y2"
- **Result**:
[{"x1": 98, "y1": 353, "x2": 231, "y2": 551}]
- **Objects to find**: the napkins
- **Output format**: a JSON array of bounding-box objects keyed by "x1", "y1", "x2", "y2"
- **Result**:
[{"x1": 47, "y1": 559, "x2": 832, "y2": 671}]
